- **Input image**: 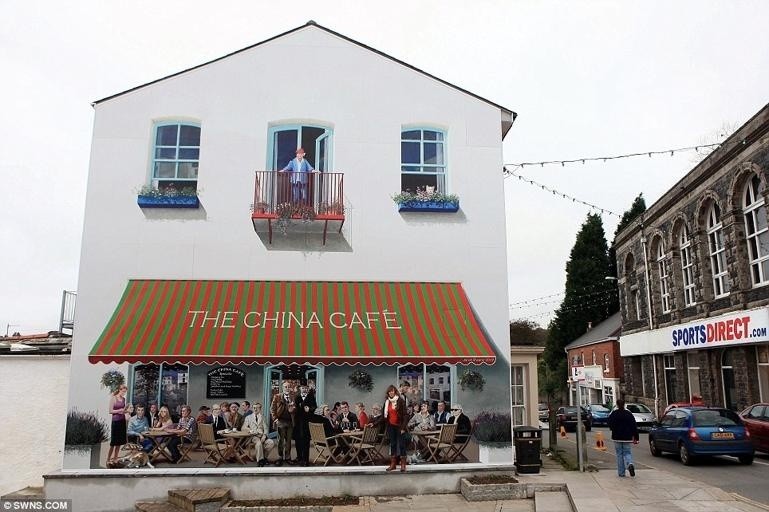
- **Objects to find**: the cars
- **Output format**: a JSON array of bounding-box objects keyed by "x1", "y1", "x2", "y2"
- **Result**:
[
  {"x1": 538, "y1": 400, "x2": 706, "y2": 433},
  {"x1": 735, "y1": 400, "x2": 769, "y2": 454},
  {"x1": 647, "y1": 405, "x2": 757, "y2": 466}
]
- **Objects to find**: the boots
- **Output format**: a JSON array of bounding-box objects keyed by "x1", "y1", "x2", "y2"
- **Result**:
[{"x1": 386, "y1": 456, "x2": 405, "y2": 471}]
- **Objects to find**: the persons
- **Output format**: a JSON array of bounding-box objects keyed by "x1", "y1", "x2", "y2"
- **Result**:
[
  {"x1": 98, "y1": 370, "x2": 474, "y2": 474},
  {"x1": 605, "y1": 395, "x2": 642, "y2": 478},
  {"x1": 276, "y1": 149, "x2": 321, "y2": 207}
]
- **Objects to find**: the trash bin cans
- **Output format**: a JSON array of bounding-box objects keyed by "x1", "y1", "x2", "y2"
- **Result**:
[{"x1": 514, "y1": 426, "x2": 542, "y2": 473}]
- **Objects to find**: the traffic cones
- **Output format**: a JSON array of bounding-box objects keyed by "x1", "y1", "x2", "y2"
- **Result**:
[
  {"x1": 590, "y1": 431, "x2": 609, "y2": 452},
  {"x1": 558, "y1": 424, "x2": 570, "y2": 439}
]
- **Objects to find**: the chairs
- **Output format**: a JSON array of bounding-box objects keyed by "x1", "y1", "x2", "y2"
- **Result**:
[{"x1": 122, "y1": 418, "x2": 481, "y2": 464}]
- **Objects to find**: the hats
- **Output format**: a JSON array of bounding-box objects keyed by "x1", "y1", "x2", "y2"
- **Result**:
[{"x1": 299, "y1": 379, "x2": 308, "y2": 387}]
